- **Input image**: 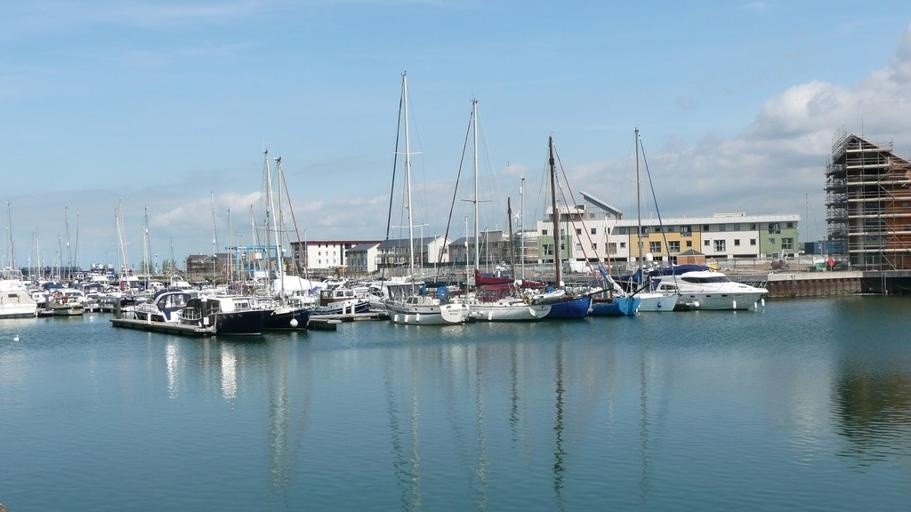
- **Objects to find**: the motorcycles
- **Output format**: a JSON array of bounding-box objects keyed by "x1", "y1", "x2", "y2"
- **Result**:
[{"x1": 770, "y1": 263, "x2": 791, "y2": 271}]
